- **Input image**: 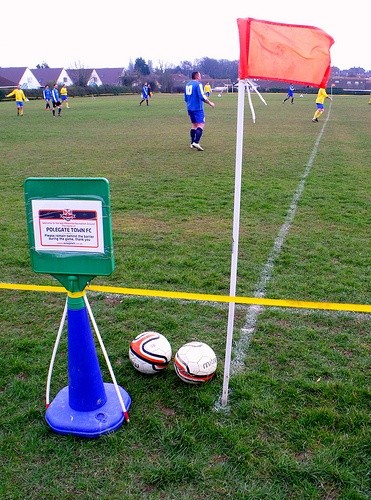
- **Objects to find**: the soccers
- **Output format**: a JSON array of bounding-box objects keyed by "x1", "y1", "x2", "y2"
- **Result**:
[
  {"x1": 130, "y1": 331, "x2": 172, "y2": 375},
  {"x1": 174, "y1": 342, "x2": 217, "y2": 386}
]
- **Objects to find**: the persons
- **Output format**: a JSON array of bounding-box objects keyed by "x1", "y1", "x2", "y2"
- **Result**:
[
  {"x1": 204, "y1": 82, "x2": 212, "y2": 98},
  {"x1": 312, "y1": 88, "x2": 333, "y2": 123},
  {"x1": 43, "y1": 82, "x2": 151, "y2": 117},
  {"x1": 283, "y1": 83, "x2": 295, "y2": 104},
  {"x1": 5, "y1": 84, "x2": 28, "y2": 116},
  {"x1": 185, "y1": 71, "x2": 215, "y2": 151}
]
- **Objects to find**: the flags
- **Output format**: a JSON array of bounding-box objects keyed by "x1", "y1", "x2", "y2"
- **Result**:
[{"x1": 236, "y1": 17, "x2": 335, "y2": 88}]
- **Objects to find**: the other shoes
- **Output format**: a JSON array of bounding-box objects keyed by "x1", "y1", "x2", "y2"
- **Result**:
[
  {"x1": 315, "y1": 119, "x2": 319, "y2": 122},
  {"x1": 312, "y1": 119, "x2": 317, "y2": 123},
  {"x1": 140, "y1": 102, "x2": 142, "y2": 106},
  {"x1": 191, "y1": 144, "x2": 194, "y2": 148},
  {"x1": 192, "y1": 142, "x2": 204, "y2": 151}
]
